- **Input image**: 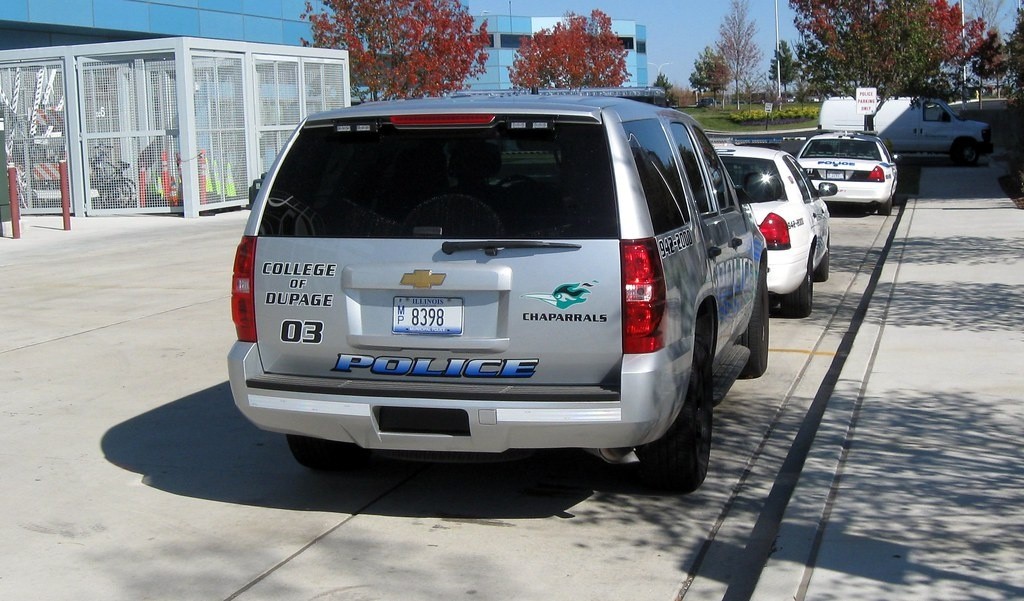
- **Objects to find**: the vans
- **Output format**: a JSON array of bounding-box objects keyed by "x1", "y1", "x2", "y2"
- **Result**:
[
  {"x1": 223, "y1": 95, "x2": 772, "y2": 496},
  {"x1": 821, "y1": 92, "x2": 993, "y2": 168}
]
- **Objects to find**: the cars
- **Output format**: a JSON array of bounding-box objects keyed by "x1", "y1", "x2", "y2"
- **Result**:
[
  {"x1": 706, "y1": 144, "x2": 840, "y2": 319},
  {"x1": 795, "y1": 131, "x2": 900, "y2": 216}
]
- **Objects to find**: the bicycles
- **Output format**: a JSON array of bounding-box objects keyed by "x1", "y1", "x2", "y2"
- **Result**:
[{"x1": 90, "y1": 142, "x2": 136, "y2": 207}]
